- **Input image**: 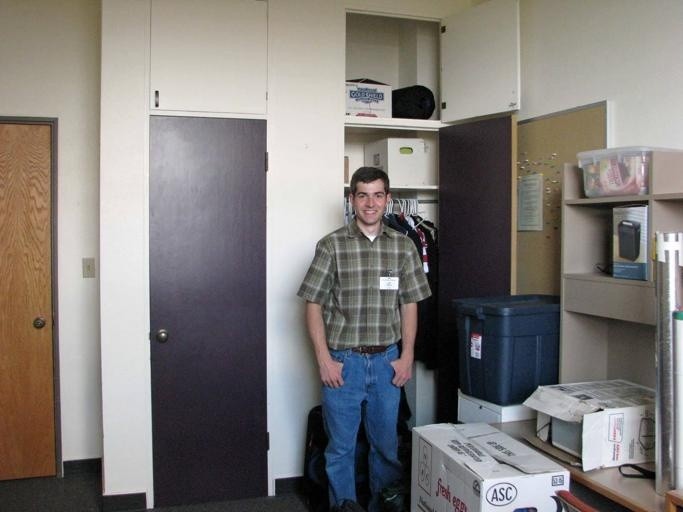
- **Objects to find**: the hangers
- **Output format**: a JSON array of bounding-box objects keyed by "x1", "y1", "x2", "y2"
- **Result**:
[{"x1": 345, "y1": 199, "x2": 428, "y2": 228}]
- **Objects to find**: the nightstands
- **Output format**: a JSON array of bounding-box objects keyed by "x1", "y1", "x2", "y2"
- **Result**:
[{"x1": 456, "y1": 390, "x2": 538, "y2": 423}]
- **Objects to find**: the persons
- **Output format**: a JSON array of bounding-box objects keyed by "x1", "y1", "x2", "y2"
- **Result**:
[{"x1": 294, "y1": 163, "x2": 432, "y2": 512}]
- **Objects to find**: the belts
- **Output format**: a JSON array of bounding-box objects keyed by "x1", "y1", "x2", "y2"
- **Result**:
[{"x1": 350, "y1": 345, "x2": 389, "y2": 354}]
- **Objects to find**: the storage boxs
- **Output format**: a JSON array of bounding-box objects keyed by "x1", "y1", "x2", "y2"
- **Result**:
[
  {"x1": 345, "y1": 83, "x2": 391, "y2": 120},
  {"x1": 410, "y1": 422, "x2": 571, "y2": 512},
  {"x1": 522, "y1": 379, "x2": 657, "y2": 473},
  {"x1": 577, "y1": 146, "x2": 653, "y2": 196},
  {"x1": 363, "y1": 138, "x2": 438, "y2": 189},
  {"x1": 450, "y1": 298, "x2": 559, "y2": 405}
]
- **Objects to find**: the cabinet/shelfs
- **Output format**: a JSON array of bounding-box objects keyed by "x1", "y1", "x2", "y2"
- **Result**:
[
  {"x1": 345, "y1": 8, "x2": 441, "y2": 193},
  {"x1": 560, "y1": 149, "x2": 682, "y2": 391}
]
